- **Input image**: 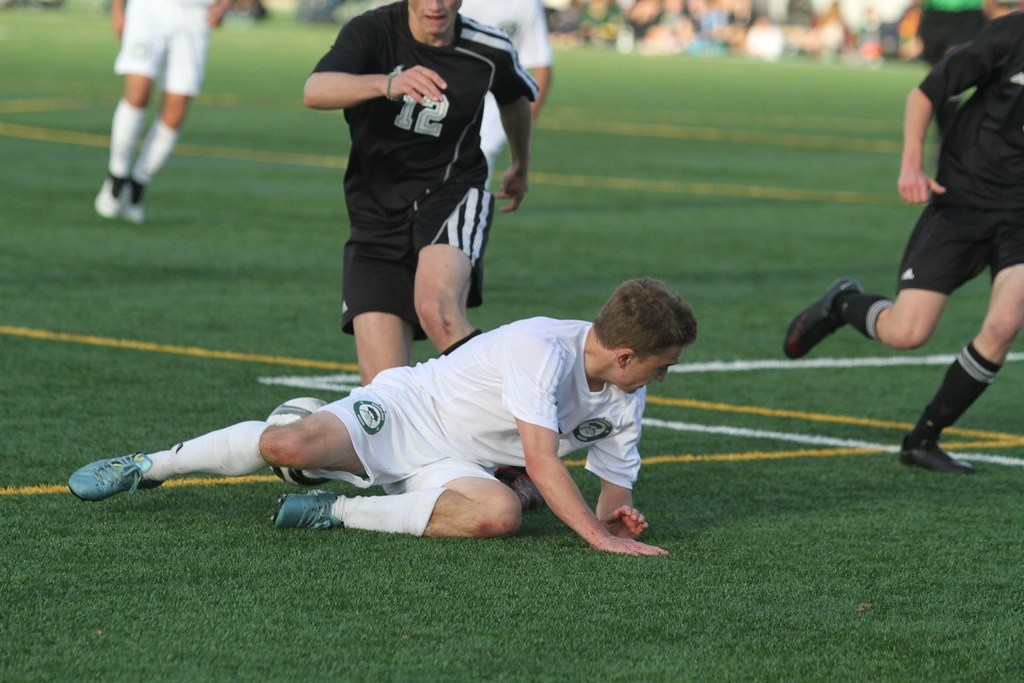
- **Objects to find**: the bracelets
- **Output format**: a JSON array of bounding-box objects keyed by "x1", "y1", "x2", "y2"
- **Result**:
[{"x1": 387, "y1": 65, "x2": 402, "y2": 101}]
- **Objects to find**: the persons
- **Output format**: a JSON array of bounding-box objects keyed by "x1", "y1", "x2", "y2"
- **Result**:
[
  {"x1": 546, "y1": 0, "x2": 1023, "y2": 131},
  {"x1": 69, "y1": 280, "x2": 698, "y2": 557},
  {"x1": 784, "y1": 13, "x2": 1024, "y2": 471},
  {"x1": 96, "y1": 0, "x2": 235, "y2": 223},
  {"x1": 303, "y1": 0, "x2": 541, "y2": 385},
  {"x1": 459, "y1": 1, "x2": 553, "y2": 186}
]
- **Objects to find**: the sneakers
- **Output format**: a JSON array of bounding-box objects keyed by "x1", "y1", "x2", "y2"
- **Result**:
[
  {"x1": 94, "y1": 175, "x2": 129, "y2": 219},
  {"x1": 120, "y1": 182, "x2": 145, "y2": 224},
  {"x1": 270, "y1": 488, "x2": 342, "y2": 530},
  {"x1": 68, "y1": 450, "x2": 166, "y2": 501},
  {"x1": 898, "y1": 434, "x2": 976, "y2": 474},
  {"x1": 783, "y1": 276, "x2": 865, "y2": 360}
]
